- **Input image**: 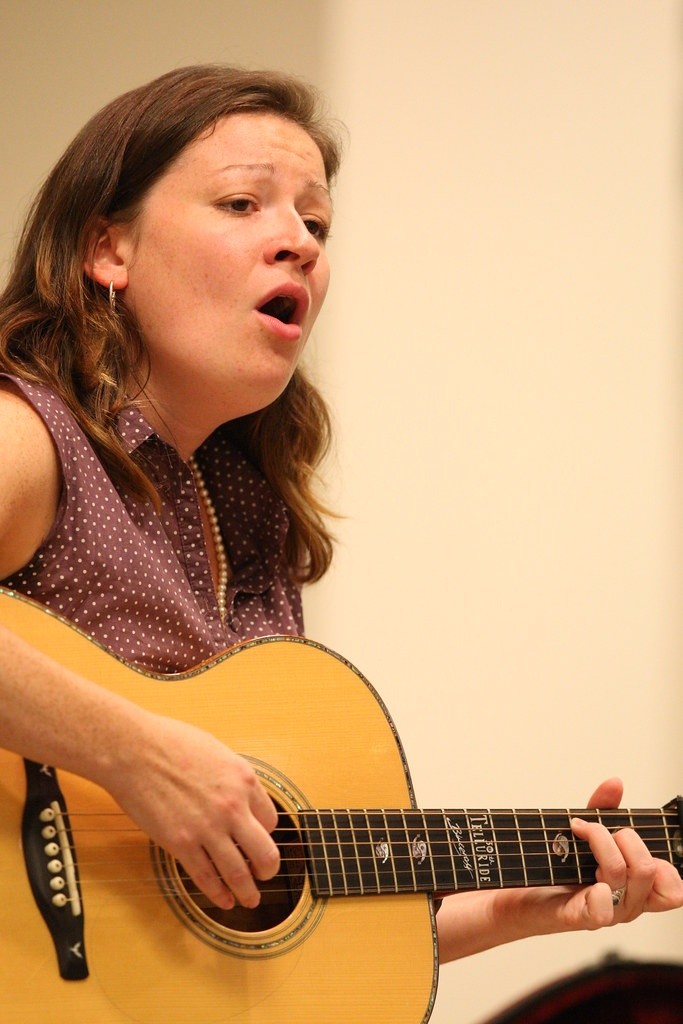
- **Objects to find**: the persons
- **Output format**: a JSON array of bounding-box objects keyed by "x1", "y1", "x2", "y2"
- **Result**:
[{"x1": 0, "y1": 64, "x2": 683, "y2": 964}]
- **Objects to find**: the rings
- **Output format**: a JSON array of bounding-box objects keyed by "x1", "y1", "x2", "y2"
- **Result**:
[{"x1": 611, "y1": 885, "x2": 626, "y2": 905}]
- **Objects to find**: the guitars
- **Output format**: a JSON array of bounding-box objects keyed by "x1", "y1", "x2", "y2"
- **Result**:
[{"x1": 3, "y1": 584, "x2": 683, "y2": 1024}]
